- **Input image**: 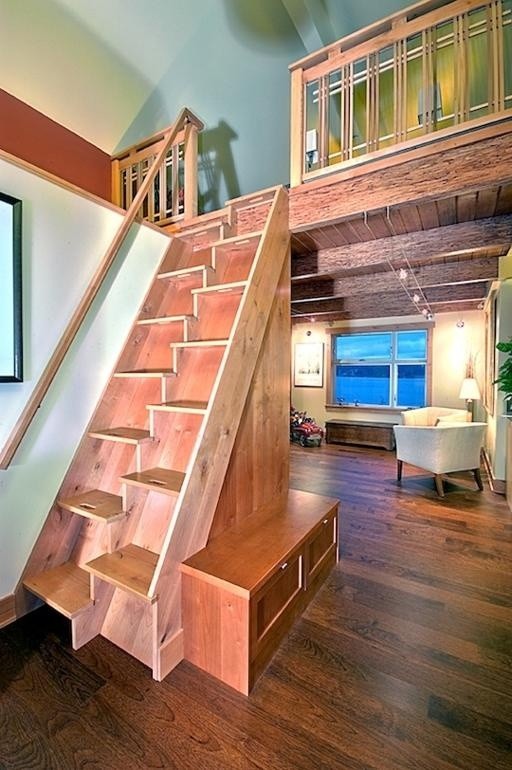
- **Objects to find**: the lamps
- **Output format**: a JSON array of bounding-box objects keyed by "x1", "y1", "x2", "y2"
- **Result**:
[{"x1": 459, "y1": 378, "x2": 481, "y2": 412}]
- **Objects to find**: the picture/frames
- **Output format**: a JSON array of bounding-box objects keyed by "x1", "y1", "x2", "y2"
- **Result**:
[{"x1": 293, "y1": 343, "x2": 324, "y2": 387}]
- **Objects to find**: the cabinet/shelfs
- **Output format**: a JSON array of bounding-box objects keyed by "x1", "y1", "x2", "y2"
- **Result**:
[
  {"x1": 178, "y1": 488, "x2": 340, "y2": 696},
  {"x1": 325, "y1": 419, "x2": 398, "y2": 451}
]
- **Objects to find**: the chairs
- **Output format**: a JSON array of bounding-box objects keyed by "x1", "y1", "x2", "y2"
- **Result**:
[{"x1": 393, "y1": 406, "x2": 488, "y2": 497}]
